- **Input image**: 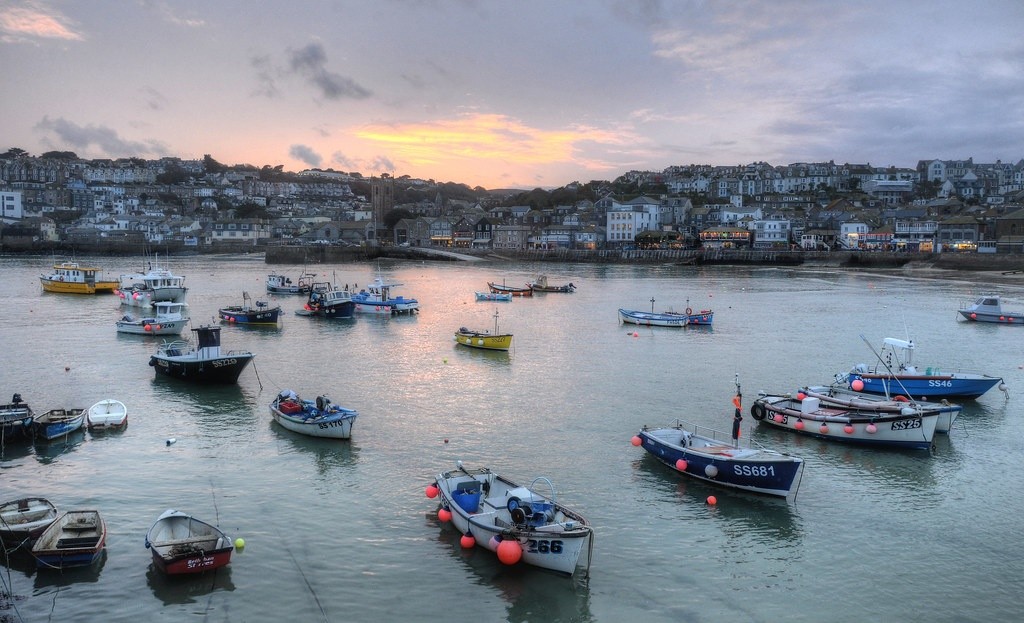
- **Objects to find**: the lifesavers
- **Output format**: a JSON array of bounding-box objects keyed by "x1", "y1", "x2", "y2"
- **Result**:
[
  {"x1": 751, "y1": 403, "x2": 766, "y2": 420},
  {"x1": 892, "y1": 395, "x2": 908, "y2": 402},
  {"x1": 686, "y1": 308, "x2": 692, "y2": 315},
  {"x1": 60, "y1": 276, "x2": 63, "y2": 281},
  {"x1": 299, "y1": 281, "x2": 304, "y2": 286}
]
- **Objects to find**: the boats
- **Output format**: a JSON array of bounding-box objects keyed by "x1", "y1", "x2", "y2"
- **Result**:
[
  {"x1": 32, "y1": 407, "x2": 87, "y2": 440},
  {"x1": 148, "y1": 314, "x2": 259, "y2": 386},
  {"x1": 38, "y1": 245, "x2": 121, "y2": 295},
  {"x1": 749, "y1": 390, "x2": 941, "y2": 451},
  {"x1": 472, "y1": 290, "x2": 513, "y2": 302},
  {"x1": 217, "y1": 290, "x2": 284, "y2": 327},
  {"x1": 664, "y1": 296, "x2": 714, "y2": 325},
  {"x1": 956, "y1": 293, "x2": 1024, "y2": 325},
  {"x1": 433, "y1": 459, "x2": 593, "y2": 577},
  {"x1": 831, "y1": 310, "x2": 1004, "y2": 402},
  {"x1": 349, "y1": 258, "x2": 419, "y2": 315},
  {"x1": 268, "y1": 388, "x2": 361, "y2": 441},
  {"x1": 115, "y1": 297, "x2": 192, "y2": 336},
  {"x1": 0, "y1": 497, "x2": 60, "y2": 555},
  {"x1": 31, "y1": 509, "x2": 108, "y2": 569},
  {"x1": 118, "y1": 241, "x2": 190, "y2": 309},
  {"x1": 453, "y1": 307, "x2": 514, "y2": 352},
  {"x1": 617, "y1": 296, "x2": 690, "y2": 327},
  {"x1": 797, "y1": 384, "x2": 964, "y2": 434},
  {"x1": 486, "y1": 278, "x2": 535, "y2": 297},
  {"x1": 264, "y1": 260, "x2": 317, "y2": 296},
  {"x1": 86, "y1": 397, "x2": 128, "y2": 430},
  {"x1": 295, "y1": 269, "x2": 358, "y2": 320},
  {"x1": 629, "y1": 372, "x2": 806, "y2": 498},
  {"x1": 144, "y1": 507, "x2": 235, "y2": 576},
  {"x1": 0, "y1": 392, "x2": 36, "y2": 440},
  {"x1": 525, "y1": 274, "x2": 578, "y2": 294}
]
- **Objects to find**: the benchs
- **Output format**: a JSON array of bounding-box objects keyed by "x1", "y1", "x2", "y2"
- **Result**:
[
  {"x1": 49, "y1": 416, "x2": 75, "y2": 419},
  {"x1": 152, "y1": 535, "x2": 219, "y2": 547},
  {"x1": 94, "y1": 413, "x2": 123, "y2": 418},
  {"x1": 0, "y1": 409, "x2": 26, "y2": 415}
]
